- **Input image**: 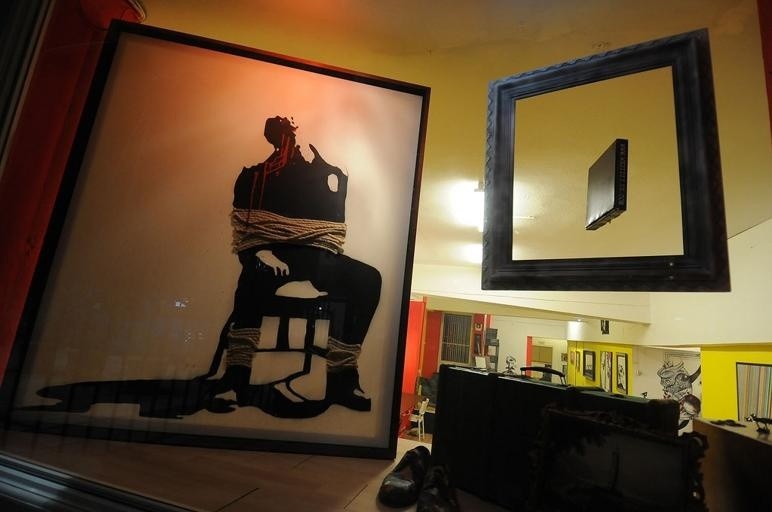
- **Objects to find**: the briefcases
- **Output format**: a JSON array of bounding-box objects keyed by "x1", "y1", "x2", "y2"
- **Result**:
[{"x1": 433, "y1": 363, "x2": 679, "y2": 511}]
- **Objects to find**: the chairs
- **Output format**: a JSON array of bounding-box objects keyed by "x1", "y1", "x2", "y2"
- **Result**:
[{"x1": 408, "y1": 398, "x2": 430, "y2": 439}]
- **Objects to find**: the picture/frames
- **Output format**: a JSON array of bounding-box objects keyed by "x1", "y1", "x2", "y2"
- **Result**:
[
  {"x1": 615, "y1": 352, "x2": 628, "y2": 395},
  {"x1": 583, "y1": 351, "x2": 595, "y2": 382},
  {"x1": 524, "y1": 401, "x2": 710, "y2": 512},
  {"x1": 0, "y1": 17, "x2": 431, "y2": 459},
  {"x1": 482, "y1": 28, "x2": 731, "y2": 292},
  {"x1": 735, "y1": 362, "x2": 771, "y2": 421}
]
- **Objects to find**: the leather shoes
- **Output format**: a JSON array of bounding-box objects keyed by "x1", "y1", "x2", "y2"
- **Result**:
[{"x1": 378, "y1": 446, "x2": 460, "y2": 512}]
